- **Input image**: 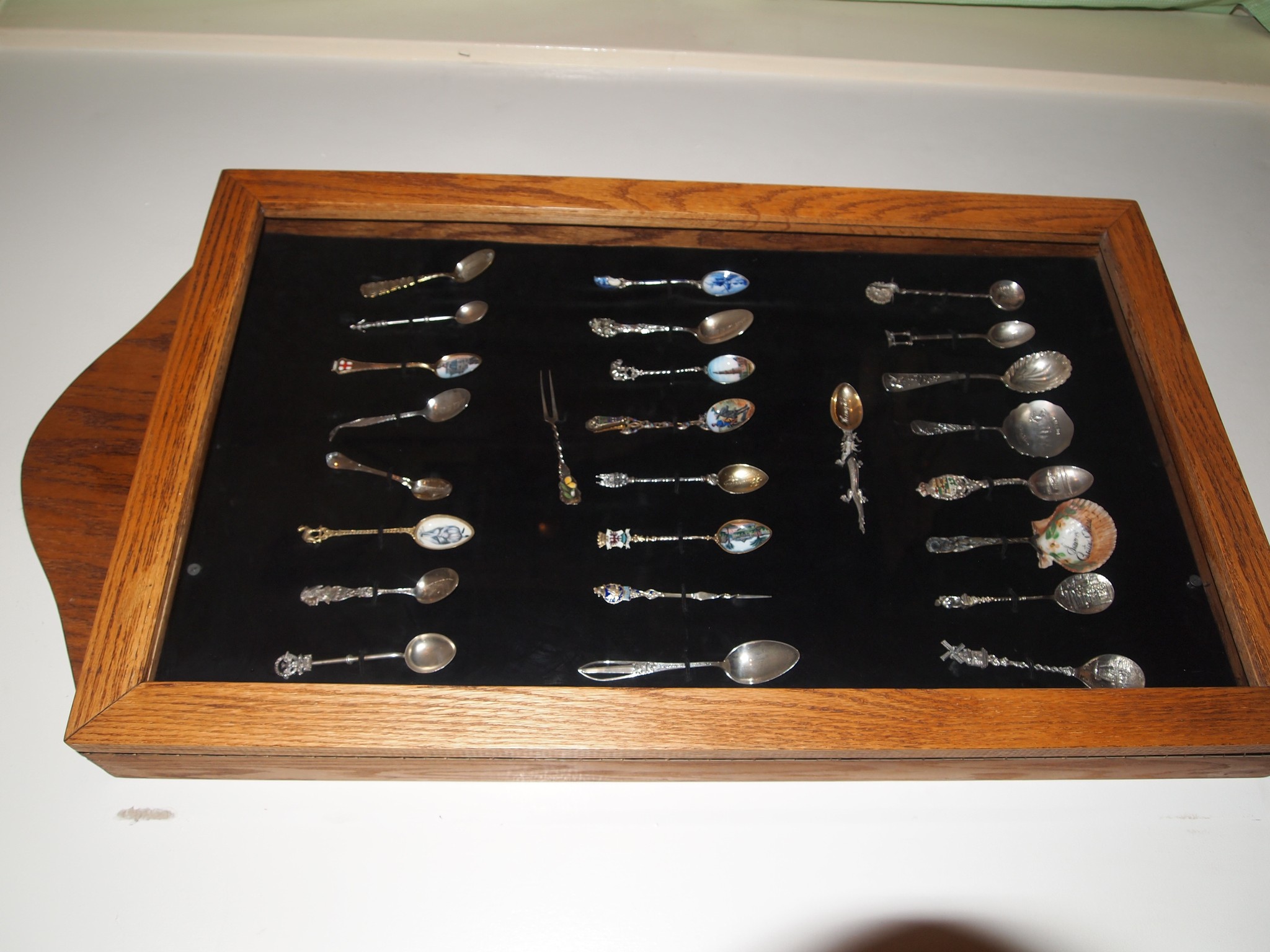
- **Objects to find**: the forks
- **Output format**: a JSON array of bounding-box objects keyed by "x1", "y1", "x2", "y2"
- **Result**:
[{"x1": 536, "y1": 368, "x2": 584, "y2": 507}]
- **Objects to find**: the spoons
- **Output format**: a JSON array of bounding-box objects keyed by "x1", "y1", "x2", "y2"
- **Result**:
[{"x1": 274, "y1": 223, "x2": 1150, "y2": 694}]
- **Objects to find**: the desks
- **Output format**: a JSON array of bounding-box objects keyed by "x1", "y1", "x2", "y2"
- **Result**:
[{"x1": 0, "y1": 0, "x2": 1267, "y2": 952}]
